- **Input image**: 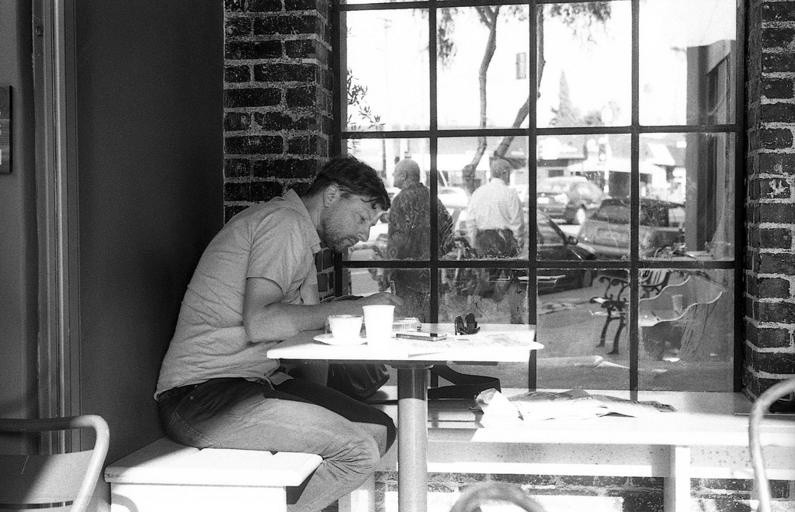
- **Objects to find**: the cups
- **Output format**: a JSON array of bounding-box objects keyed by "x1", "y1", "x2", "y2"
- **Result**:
[
  {"x1": 362, "y1": 304, "x2": 397, "y2": 345},
  {"x1": 328, "y1": 314, "x2": 364, "y2": 338}
]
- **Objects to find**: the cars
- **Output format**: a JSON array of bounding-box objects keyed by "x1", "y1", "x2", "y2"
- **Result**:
[
  {"x1": 518, "y1": 175, "x2": 614, "y2": 225},
  {"x1": 350, "y1": 169, "x2": 469, "y2": 250},
  {"x1": 440, "y1": 204, "x2": 599, "y2": 297},
  {"x1": 576, "y1": 197, "x2": 685, "y2": 261}
]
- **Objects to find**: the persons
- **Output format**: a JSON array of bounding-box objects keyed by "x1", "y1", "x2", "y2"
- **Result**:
[
  {"x1": 464, "y1": 158, "x2": 527, "y2": 324},
  {"x1": 151, "y1": 156, "x2": 406, "y2": 510},
  {"x1": 378, "y1": 158, "x2": 457, "y2": 323}
]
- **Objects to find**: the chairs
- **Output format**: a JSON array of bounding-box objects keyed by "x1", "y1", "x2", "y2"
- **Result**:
[{"x1": 590, "y1": 248, "x2": 725, "y2": 359}]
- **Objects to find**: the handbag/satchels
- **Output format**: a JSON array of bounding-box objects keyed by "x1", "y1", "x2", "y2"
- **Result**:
[{"x1": 328, "y1": 363, "x2": 389, "y2": 401}]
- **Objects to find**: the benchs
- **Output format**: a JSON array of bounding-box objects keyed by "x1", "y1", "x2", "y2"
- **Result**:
[{"x1": 103, "y1": 384, "x2": 399, "y2": 510}]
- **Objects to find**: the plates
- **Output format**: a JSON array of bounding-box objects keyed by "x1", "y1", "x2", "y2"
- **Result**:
[{"x1": 311, "y1": 333, "x2": 367, "y2": 346}]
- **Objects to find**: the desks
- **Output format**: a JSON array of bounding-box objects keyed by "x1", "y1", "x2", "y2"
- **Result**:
[{"x1": 267, "y1": 321, "x2": 536, "y2": 511}]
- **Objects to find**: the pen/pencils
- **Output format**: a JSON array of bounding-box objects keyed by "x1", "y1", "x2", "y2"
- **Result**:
[
  {"x1": 407, "y1": 331, "x2": 438, "y2": 337},
  {"x1": 390, "y1": 281, "x2": 396, "y2": 296}
]
